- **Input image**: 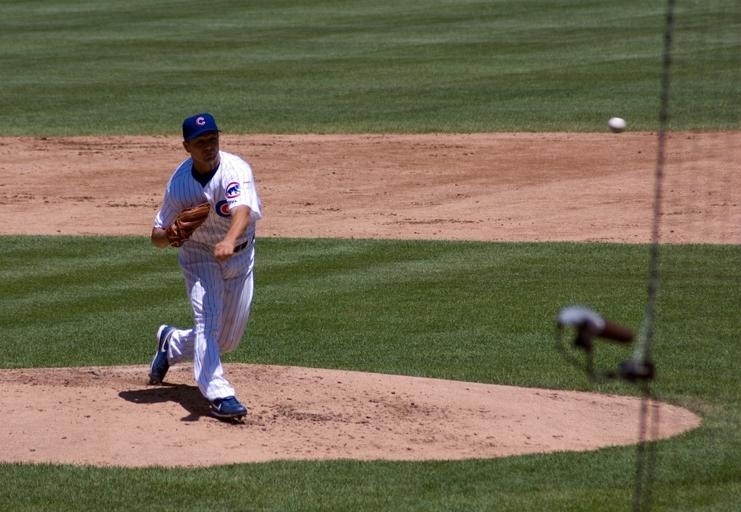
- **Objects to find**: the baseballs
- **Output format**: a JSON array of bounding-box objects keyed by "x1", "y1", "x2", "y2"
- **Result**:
[{"x1": 608, "y1": 117, "x2": 627, "y2": 132}]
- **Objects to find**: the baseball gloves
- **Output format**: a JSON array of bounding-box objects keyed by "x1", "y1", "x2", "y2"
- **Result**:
[{"x1": 167, "y1": 202, "x2": 209, "y2": 247}]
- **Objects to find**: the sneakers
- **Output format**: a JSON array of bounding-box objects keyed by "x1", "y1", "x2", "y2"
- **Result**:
[
  {"x1": 150, "y1": 324, "x2": 175, "y2": 382},
  {"x1": 209, "y1": 395, "x2": 248, "y2": 420}
]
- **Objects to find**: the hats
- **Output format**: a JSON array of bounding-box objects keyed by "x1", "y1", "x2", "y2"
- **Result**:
[{"x1": 183, "y1": 113, "x2": 222, "y2": 142}]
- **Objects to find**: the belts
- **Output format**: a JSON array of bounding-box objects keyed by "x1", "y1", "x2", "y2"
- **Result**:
[{"x1": 234, "y1": 236, "x2": 255, "y2": 253}]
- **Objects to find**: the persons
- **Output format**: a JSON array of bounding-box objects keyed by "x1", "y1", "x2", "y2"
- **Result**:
[{"x1": 147, "y1": 114, "x2": 262, "y2": 418}]
